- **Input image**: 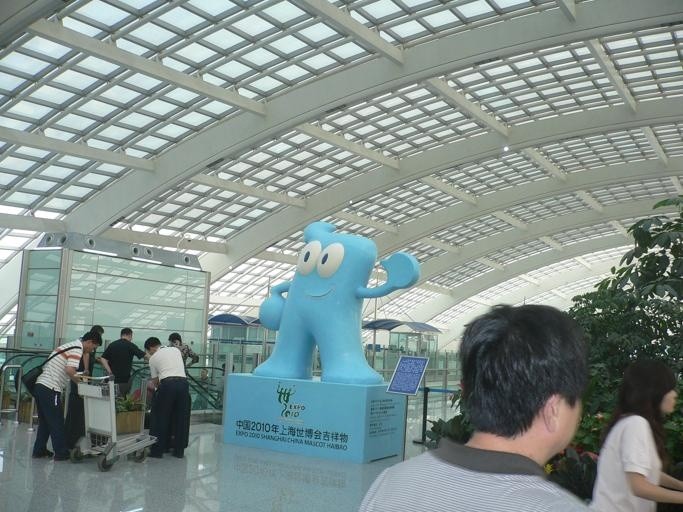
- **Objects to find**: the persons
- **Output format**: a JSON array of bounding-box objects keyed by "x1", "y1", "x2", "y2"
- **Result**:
[
  {"x1": 193, "y1": 368, "x2": 209, "y2": 411},
  {"x1": 100, "y1": 325, "x2": 148, "y2": 396},
  {"x1": 28, "y1": 331, "x2": 100, "y2": 461},
  {"x1": 143, "y1": 337, "x2": 190, "y2": 460},
  {"x1": 359, "y1": 304, "x2": 602, "y2": 512},
  {"x1": 169, "y1": 332, "x2": 199, "y2": 370},
  {"x1": 220, "y1": 362, "x2": 234, "y2": 378},
  {"x1": 62, "y1": 325, "x2": 103, "y2": 439},
  {"x1": 588, "y1": 357, "x2": 682, "y2": 512}
]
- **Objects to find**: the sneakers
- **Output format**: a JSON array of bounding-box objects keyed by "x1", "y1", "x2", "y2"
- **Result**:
[
  {"x1": 171, "y1": 449, "x2": 184, "y2": 458},
  {"x1": 32, "y1": 449, "x2": 53, "y2": 459},
  {"x1": 146, "y1": 449, "x2": 163, "y2": 458},
  {"x1": 54, "y1": 449, "x2": 73, "y2": 461}
]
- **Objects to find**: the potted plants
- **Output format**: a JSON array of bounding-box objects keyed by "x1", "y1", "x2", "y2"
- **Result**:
[
  {"x1": 114, "y1": 392, "x2": 148, "y2": 434},
  {"x1": 16, "y1": 392, "x2": 37, "y2": 424}
]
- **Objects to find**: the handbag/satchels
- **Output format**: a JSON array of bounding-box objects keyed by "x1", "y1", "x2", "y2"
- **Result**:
[{"x1": 21, "y1": 366, "x2": 43, "y2": 397}]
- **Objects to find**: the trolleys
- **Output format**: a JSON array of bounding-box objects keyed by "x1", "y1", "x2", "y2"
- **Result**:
[{"x1": 73, "y1": 372, "x2": 158, "y2": 472}]
